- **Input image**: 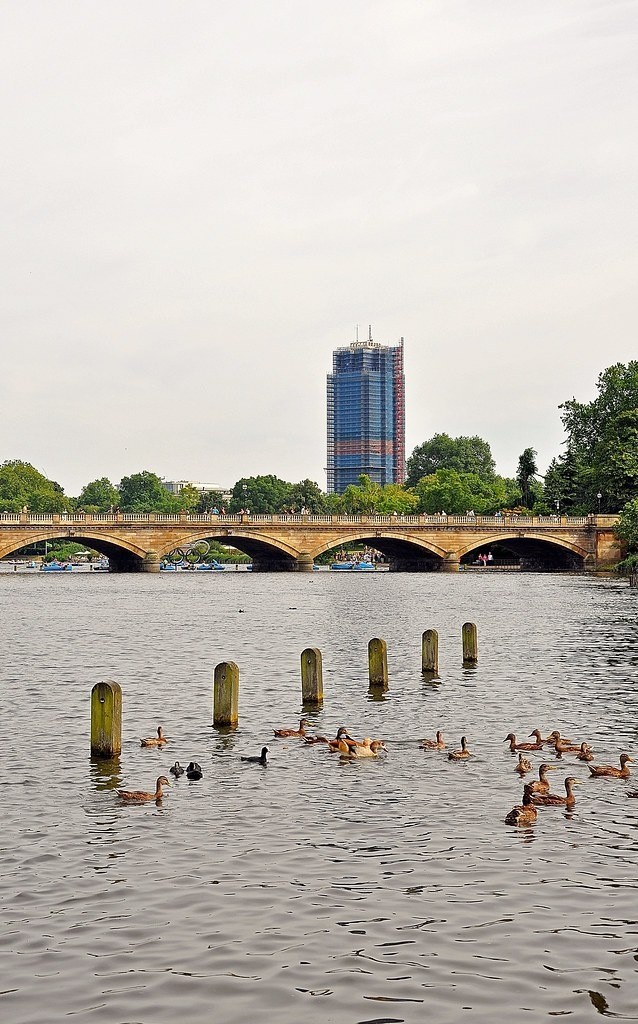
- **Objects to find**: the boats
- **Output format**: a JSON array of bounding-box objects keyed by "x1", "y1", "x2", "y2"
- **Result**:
[
  {"x1": 313, "y1": 565, "x2": 319, "y2": 570},
  {"x1": 67, "y1": 563, "x2": 83, "y2": 566},
  {"x1": 360, "y1": 563, "x2": 373, "y2": 568},
  {"x1": 40, "y1": 565, "x2": 72, "y2": 570},
  {"x1": 331, "y1": 564, "x2": 353, "y2": 569},
  {"x1": 26, "y1": 564, "x2": 37, "y2": 568},
  {"x1": 354, "y1": 565, "x2": 362, "y2": 570},
  {"x1": 247, "y1": 566, "x2": 253, "y2": 570},
  {"x1": 94, "y1": 563, "x2": 109, "y2": 570},
  {"x1": 160, "y1": 564, "x2": 174, "y2": 570},
  {"x1": 197, "y1": 564, "x2": 224, "y2": 570},
  {"x1": 181, "y1": 566, "x2": 196, "y2": 570},
  {"x1": 172, "y1": 562, "x2": 184, "y2": 565}
]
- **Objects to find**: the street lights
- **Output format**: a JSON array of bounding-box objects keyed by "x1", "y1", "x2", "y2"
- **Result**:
[
  {"x1": 597, "y1": 490, "x2": 603, "y2": 513},
  {"x1": 242, "y1": 484, "x2": 247, "y2": 512}
]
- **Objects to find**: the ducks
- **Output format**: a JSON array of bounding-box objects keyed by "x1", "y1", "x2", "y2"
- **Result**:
[
  {"x1": 139, "y1": 726, "x2": 168, "y2": 747},
  {"x1": 448, "y1": 736, "x2": 473, "y2": 761},
  {"x1": 113, "y1": 776, "x2": 172, "y2": 801},
  {"x1": 418, "y1": 731, "x2": 445, "y2": 752},
  {"x1": 170, "y1": 761, "x2": 203, "y2": 779},
  {"x1": 272, "y1": 718, "x2": 388, "y2": 759},
  {"x1": 502, "y1": 727, "x2": 635, "y2": 828},
  {"x1": 241, "y1": 746, "x2": 269, "y2": 766}
]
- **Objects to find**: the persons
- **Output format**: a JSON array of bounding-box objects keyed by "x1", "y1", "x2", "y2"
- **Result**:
[
  {"x1": 335, "y1": 544, "x2": 388, "y2": 569},
  {"x1": 479, "y1": 551, "x2": 494, "y2": 566},
  {"x1": 160, "y1": 556, "x2": 220, "y2": 569},
  {"x1": 18, "y1": 550, "x2": 109, "y2": 569},
  {"x1": 1, "y1": 503, "x2": 577, "y2": 522}
]
